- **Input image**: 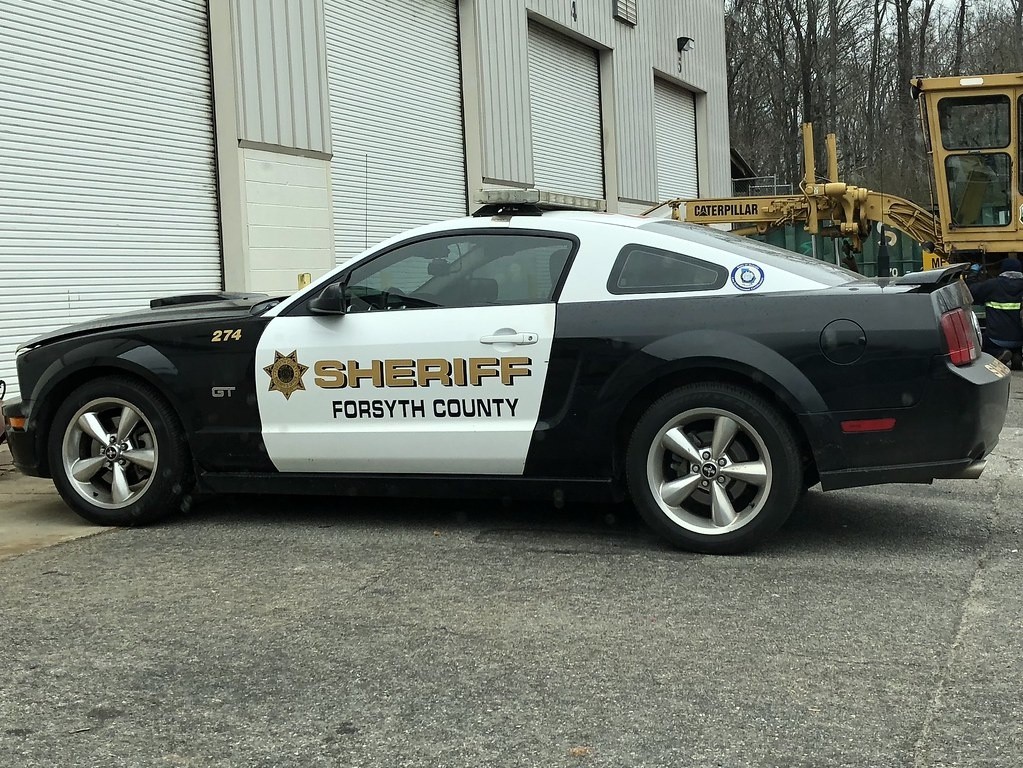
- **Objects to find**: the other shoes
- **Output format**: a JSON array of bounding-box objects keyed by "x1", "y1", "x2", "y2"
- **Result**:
[
  {"x1": 1011, "y1": 355, "x2": 1022, "y2": 368},
  {"x1": 998, "y1": 350, "x2": 1012, "y2": 364}
]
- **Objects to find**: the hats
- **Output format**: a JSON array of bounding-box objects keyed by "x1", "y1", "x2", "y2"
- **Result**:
[{"x1": 999, "y1": 258, "x2": 1022, "y2": 272}]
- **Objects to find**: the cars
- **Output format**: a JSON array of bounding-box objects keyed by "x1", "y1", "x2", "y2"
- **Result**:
[{"x1": 5, "y1": 184, "x2": 1010, "y2": 549}]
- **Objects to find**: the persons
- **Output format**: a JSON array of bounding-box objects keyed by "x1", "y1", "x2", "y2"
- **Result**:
[{"x1": 967, "y1": 256, "x2": 1023, "y2": 371}]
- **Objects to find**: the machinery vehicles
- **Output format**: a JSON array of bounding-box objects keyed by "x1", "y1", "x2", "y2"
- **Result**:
[{"x1": 641, "y1": 67, "x2": 1020, "y2": 320}]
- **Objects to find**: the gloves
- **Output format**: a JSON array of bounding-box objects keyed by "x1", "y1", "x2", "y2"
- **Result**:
[{"x1": 970, "y1": 264, "x2": 982, "y2": 271}]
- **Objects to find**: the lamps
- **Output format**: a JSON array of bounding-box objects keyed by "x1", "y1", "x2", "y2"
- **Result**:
[{"x1": 677, "y1": 36, "x2": 695, "y2": 61}]
